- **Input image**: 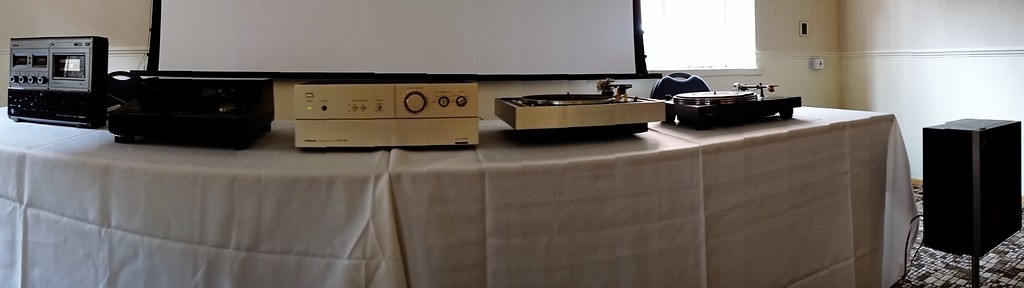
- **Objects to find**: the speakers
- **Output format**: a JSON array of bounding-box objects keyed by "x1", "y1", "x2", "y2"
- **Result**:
[{"x1": 923, "y1": 118, "x2": 1023, "y2": 257}]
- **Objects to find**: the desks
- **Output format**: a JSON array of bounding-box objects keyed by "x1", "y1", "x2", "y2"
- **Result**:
[{"x1": 2, "y1": 106, "x2": 921, "y2": 287}]
